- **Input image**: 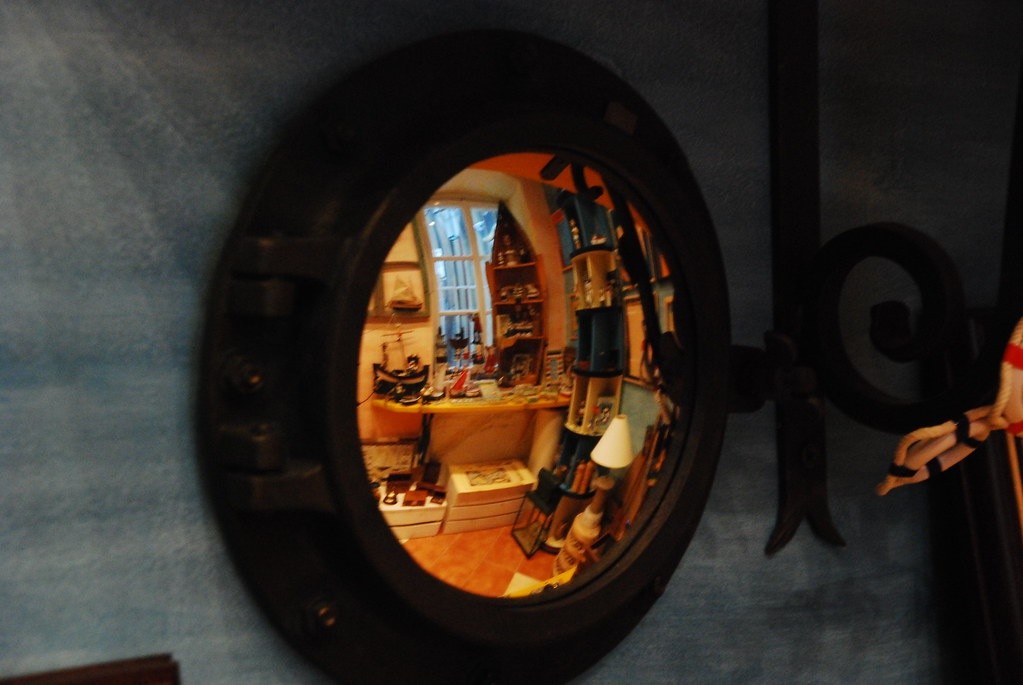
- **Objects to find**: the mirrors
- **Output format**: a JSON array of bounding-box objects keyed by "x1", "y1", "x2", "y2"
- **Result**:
[{"x1": 199, "y1": 33, "x2": 736, "y2": 685}]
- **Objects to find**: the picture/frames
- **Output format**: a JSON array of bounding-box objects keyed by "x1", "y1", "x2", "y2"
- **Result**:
[
  {"x1": 662, "y1": 296, "x2": 676, "y2": 333},
  {"x1": 621, "y1": 293, "x2": 658, "y2": 389}
]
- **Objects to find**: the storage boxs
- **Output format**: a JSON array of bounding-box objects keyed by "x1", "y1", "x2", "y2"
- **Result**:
[{"x1": 376, "y1": 459, "x2": 534, "y2": 539}]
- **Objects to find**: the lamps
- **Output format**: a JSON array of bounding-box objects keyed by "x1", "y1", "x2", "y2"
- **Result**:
[{"x1": 553, "y1": 414, "x2": 633, "y2": 580}]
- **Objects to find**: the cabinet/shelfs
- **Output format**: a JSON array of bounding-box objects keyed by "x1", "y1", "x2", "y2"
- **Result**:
[
  {"x1": 535, "y1": 183, "x2": 628, "y2": 556},
  {"x1": 484, "y1": 200, "x2": 547, "y2": 388},
  {"x1": 365, "y1": 218, "x2": 432, "y2": 324}
]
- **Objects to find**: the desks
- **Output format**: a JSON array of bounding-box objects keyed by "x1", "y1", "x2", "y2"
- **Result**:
[{"x1": 372, "y1": 383, "x2": 571, "y2": 463}]
